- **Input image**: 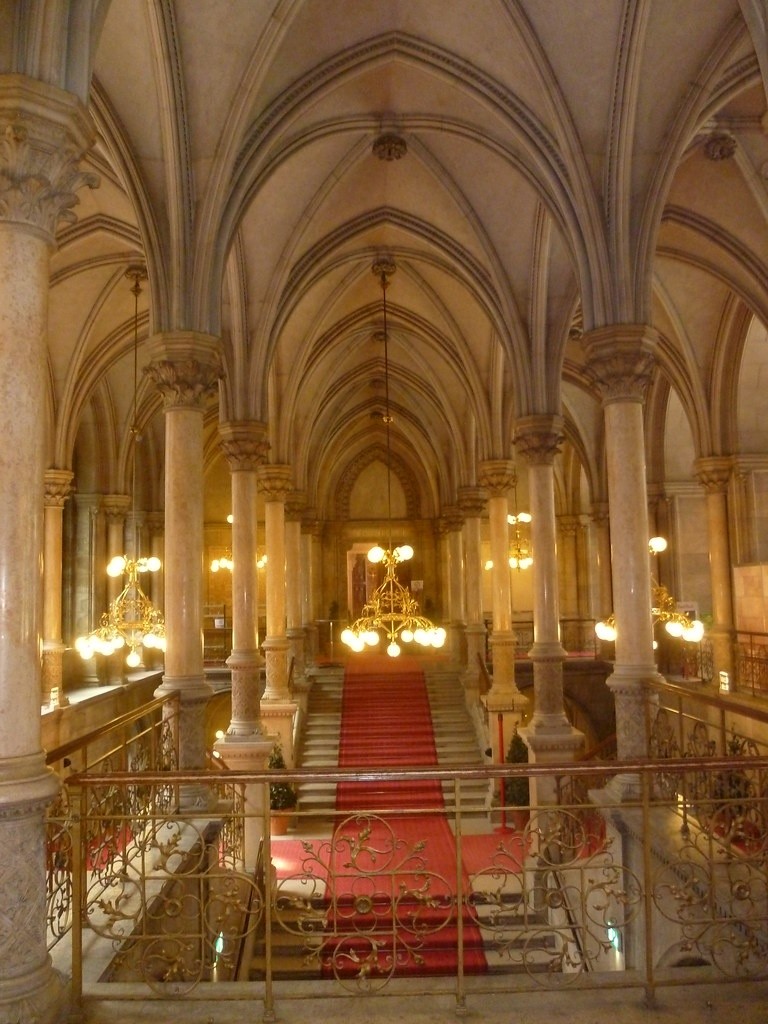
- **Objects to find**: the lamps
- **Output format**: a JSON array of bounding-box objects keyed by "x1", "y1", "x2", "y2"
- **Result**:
[
  {"x1": 507, "y1": 483, "x2": 533, "y2": 571},
  {"x1": 593, "y1": 499, "x2": 702, "y2": 652},
  {"x1": 72, "y1": 265, "x2": 171, "y2": 667},
  {"x1": 342, "y1": 258, "x2": 448, "y2": 659}
]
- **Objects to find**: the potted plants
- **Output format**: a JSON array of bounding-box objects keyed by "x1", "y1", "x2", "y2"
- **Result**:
[
  {"x1": 492, "y1": 721, "x2": 529, "y2": 833},
  {"x1": 267, "y1": 734, "x2": 297, "y2": 836}
]
